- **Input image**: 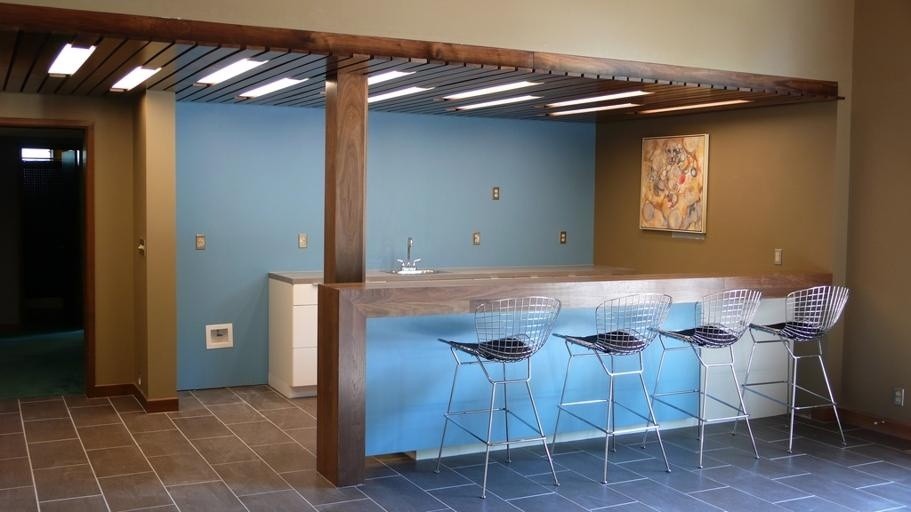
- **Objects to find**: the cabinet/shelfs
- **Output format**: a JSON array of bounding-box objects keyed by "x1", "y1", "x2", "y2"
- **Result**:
[{"x1": 266, "y1": 278, "x2": 324, "y2": 399}]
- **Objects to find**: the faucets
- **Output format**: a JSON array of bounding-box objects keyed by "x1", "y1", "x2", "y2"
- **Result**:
[{"x1": 407, "y1": 237, "x2": 414, "y2": 263}]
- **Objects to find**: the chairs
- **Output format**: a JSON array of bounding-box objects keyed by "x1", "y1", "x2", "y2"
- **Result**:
[
  {"x1": 641, "y1": 288, "x2": 763, "y2": 470},
  {"x1": 432, "y1": 296, "x2": 561, "y2": 500},
  {"x1": 731, "y1": 284, "x2": 850, "y2": 455},
  {"x1": 548, "y1": 293, "x2": 673, "y2": 486}
]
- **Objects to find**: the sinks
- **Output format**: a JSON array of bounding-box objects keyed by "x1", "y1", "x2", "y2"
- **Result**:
[{"x1": 383, "y1": 270, "x2": 449, "y2": 275}]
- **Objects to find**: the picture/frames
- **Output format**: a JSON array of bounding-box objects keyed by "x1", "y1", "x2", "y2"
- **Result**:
[{"x1": 638, "y1": 133, "x2": 710, "y2": 234}]
